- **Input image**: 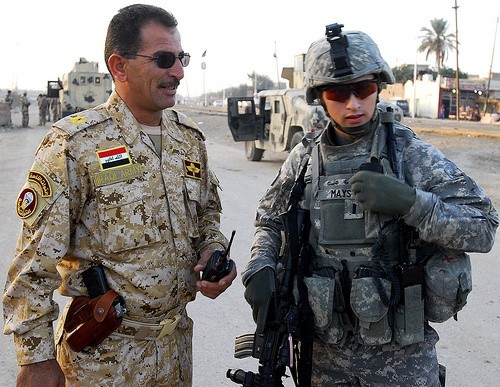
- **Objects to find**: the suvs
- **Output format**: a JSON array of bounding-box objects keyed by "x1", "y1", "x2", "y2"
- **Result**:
[{"x1": 227, "y1": 54, "x2": 403, "y2": 161}]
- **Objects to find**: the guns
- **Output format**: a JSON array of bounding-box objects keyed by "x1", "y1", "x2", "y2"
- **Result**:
[
  {"x1": 386, "y1": 106, "x2": 426, "y2": 318},
  {"x1": 80, "y1": 264, "x2": 126, "y2": 317},
  {"x1": 226, "y1": 208, "x2": 312, "y2": 387}
]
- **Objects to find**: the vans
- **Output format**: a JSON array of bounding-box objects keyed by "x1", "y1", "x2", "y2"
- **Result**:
[{"x1": 47, "y1": 56, "x2": 112, "y2": 120}]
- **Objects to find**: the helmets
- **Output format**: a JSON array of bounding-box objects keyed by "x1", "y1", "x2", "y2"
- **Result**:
[{"x1": 301, "y1": 21, "x2": 395, "y2": 102}]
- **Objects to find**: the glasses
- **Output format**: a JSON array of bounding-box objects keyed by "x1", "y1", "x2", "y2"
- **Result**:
[
  {"x1": 321, "y1": 82, "x2": 378, "y2": 103},
  {"x1": 118, "y1": 50, "x2": 191, "y2": 69}
]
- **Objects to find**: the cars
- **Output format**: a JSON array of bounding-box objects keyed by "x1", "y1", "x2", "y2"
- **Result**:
[{"x1": 213, "y1": 100, "x2": 223, "y2": 107}]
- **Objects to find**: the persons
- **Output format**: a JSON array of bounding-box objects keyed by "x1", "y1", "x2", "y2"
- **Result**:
[
  {"x1": 460, "y1": 104, "x2": 481, "y2": 121},
  {"x1": 20, "y1": 92, "x2": 31, "y2": 127},
  {"x1": 5, "y1": 91, "x2": 13, "y2": 126},
  {"x1": 441, "y1": 104, "x2": 446, "y2": 119},
  {"x1": 242, "y1": 30, "x2": 500, "y2": 387},
  {"x1": 37, "y1": 94, "x2": 57, "y2": 126},
  {"x1": 3, "y1": 4, "x2": 237, "y2": 387}
]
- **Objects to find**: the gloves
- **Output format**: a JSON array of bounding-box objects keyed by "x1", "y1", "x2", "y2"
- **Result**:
[
  {"x1": 244, "y1": 267, "x2": 284, "y2": 334},
  {"x1": 347, "y1": 170, "x2": 417, "y2": 217}
]
